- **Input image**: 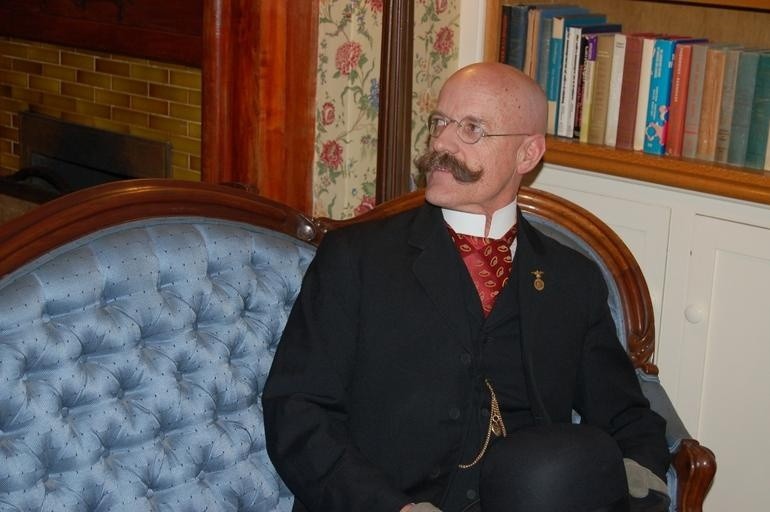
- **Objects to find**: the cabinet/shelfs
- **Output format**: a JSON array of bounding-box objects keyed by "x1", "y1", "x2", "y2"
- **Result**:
[{"x1": 480, "y1": 0, "x2": 768, "y2": 203}]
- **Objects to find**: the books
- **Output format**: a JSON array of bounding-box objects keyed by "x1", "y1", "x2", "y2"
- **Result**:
[{"x1": 497, "y1": 4, "x2": 770, "y2": 171}]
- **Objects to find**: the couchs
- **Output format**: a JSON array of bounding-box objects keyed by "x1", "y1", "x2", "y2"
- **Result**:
[{"x1": 0, "y1": 176, "x2": 718, "y2": 512}]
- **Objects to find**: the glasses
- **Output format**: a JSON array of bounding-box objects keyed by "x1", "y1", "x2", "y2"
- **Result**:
[{"x1": 426, "y1": 112, "x2": 531, "y2": 144}]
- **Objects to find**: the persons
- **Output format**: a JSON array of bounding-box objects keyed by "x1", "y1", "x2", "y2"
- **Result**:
[{"x1": 261, "y1": 63, "x2": 672, "y2": 512}]
